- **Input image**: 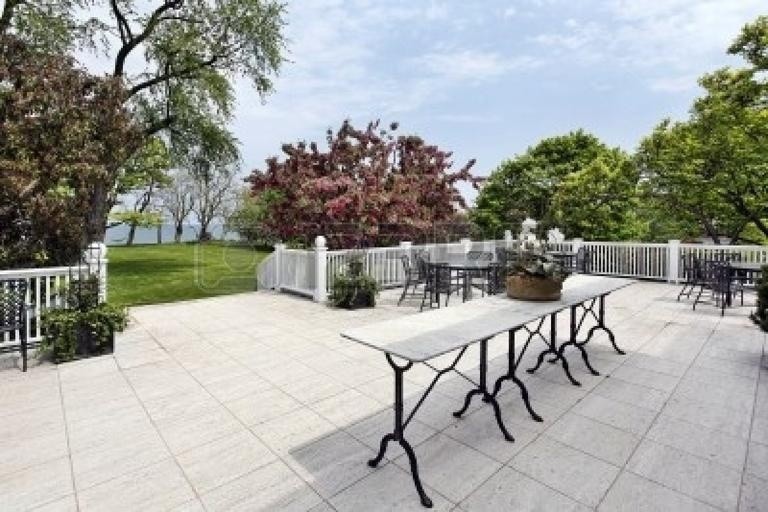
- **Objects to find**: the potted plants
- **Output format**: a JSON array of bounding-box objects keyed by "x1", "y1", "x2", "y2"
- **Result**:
[
  {"x1": 328, "y1": 258, "x2": 376, "y2": 309},
  {"x1": 33, "y1": 271, "x2": 130, "y2": 364}
]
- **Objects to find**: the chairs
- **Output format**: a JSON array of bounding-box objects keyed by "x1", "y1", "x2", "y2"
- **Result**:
[
  {"x1": 396, "y1": 241, "x2": 592, "y2": 312},
  {"x1": 678, "y1": 253, "x2": 743, "y2": 316},
  {"x1": 0, "y1": 278, "x2": 27, "y2": 371}
]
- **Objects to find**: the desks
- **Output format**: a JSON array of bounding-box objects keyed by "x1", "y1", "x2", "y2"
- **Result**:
[
  {"x1": 340, "y1": 274, "x2": 638, "y2": 508},
  {"x1": 730, "y1": 262, "x2": 768, "y2": 306}
]
